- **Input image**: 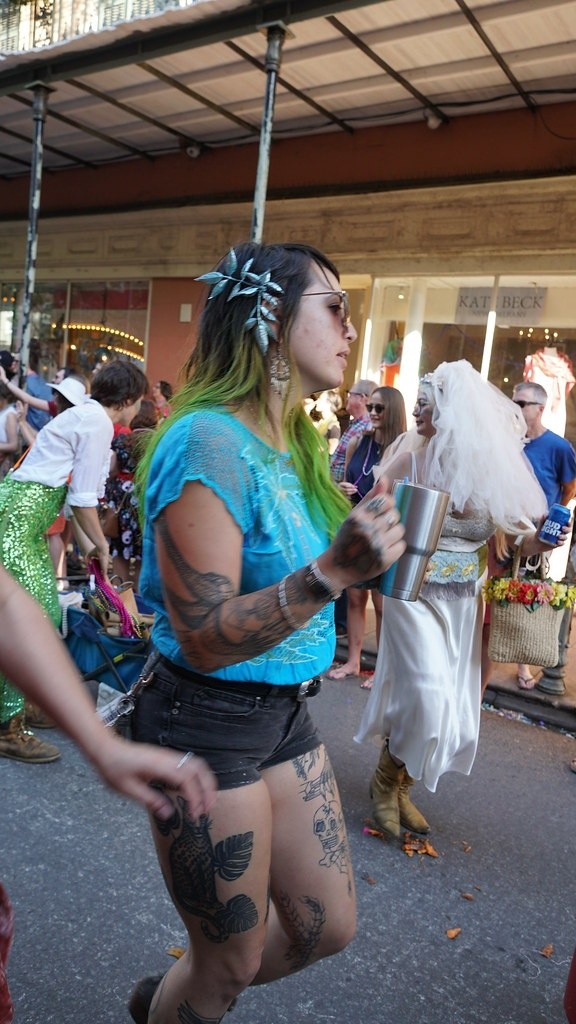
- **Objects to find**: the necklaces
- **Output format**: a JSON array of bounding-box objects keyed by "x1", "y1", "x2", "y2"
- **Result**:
[
  {"x1": 246, "y1": 401, "x2": 292, "y2": 467},
  {"x1": 363, "y1": 437, "x2": 381, "y2": 476},
  {"x1": 377, "y1": 444, "x2": 380, "y2": 454}
]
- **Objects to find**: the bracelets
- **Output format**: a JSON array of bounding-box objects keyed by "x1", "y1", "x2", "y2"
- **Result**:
[{"x1": 278, "y1": 574, "x2": 309, "y2": 629}]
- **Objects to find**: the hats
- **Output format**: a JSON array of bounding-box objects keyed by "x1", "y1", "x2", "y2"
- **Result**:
[{"x1": 46, "y1": 377, "x2": 87, "y2": 407}]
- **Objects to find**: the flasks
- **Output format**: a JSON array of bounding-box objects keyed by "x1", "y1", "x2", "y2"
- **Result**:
[{"x1": 374, "y1": 480, "x2": 451, "y2": 604}]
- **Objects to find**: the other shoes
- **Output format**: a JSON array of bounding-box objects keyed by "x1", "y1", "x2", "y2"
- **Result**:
[{"x1": 129, "y1": 970, "x2": 165, "y2": 1024}]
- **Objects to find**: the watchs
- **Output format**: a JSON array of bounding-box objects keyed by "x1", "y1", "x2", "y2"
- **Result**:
[{"x1": 305, "y1": 561, "x2": 342, "y2": 603}]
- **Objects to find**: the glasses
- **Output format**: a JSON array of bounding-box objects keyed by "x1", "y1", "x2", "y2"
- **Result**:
[
  {"x1": 412, "y1": 401, "x2": 429, "y2": 413},
  {"x1": 365, "y1": 404, "x2": 386, "y2": 414},
  {"x1": 513, "y1": 399, "x2": 543, "y2": 410},
  {"x1": 347, "y1": 391, "x2": 368, "y2": 397},
  {"x1": 280, "y1": 292, "x2": 351, "y2": 324}
]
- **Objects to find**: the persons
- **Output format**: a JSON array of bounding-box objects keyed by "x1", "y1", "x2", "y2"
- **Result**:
[
  {"x1": 373, "y1": 358, "x2": 550, "y2": 834},
  {"x1": 0, "y1": 347, "x2": 171, "y2": 764},
  {"x1": 116, "y1": 243, "x2": 408, "y2": 1024},
  {"x1": 328, "y1": 381, "x2": 576, "y2": 687},
  {"x1": 524, "y1": 347, "x2": 575, "y2": 438},
  {"x1": 0, "y1": 568, "x2": 216, "y2": 1024}
]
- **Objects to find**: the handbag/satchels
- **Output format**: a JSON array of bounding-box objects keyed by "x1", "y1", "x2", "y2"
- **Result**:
[
  {"x1": 88, "y1": 573, "x2": 142, "y2": 634},
  {"x1": 110, "y1": 697, "x2": 134, "y2": 741},
  {"x1": 97, "y1": 507, "x2": 120, "y2": 537},
  {"x1": 487, "y1": 533, "x2": 567, "y2": 666}
]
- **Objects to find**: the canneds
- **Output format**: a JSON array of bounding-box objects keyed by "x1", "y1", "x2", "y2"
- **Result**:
[{"x1": 537, "y1": 503, "x2": 572, "y2": 546}]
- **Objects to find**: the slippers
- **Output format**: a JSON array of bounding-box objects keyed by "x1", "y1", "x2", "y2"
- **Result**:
[
  {"x1": 361, "y1": 676, "x2": 375, "y2": 691},
  {"x1": 325, "y1": 667, "x2": 358, "y2": 681},
  {"x1": 516, "y1": 674, "x2": 534, "y2": 689}
]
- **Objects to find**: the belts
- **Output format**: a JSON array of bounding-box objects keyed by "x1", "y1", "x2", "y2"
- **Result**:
[{"x1": 156, "y1": 654, "x2": 323, "y2": 703}]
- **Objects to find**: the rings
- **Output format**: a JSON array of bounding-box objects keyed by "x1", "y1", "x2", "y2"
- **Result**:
[{"x1": 177, "y1": 752, "x2": 190, "y2": 770}]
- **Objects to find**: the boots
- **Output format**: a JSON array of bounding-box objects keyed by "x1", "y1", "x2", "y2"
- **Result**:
[
  {"x1": 19, "y1": 700, "x2": 54, "y2": 728},
  {"x1": 398, "y1": 771, "x2": 430, "y2": 835},
  {"x1": 368, "y1": 738, "x2": 406, "y2": 837},
  {"x1": 0, "y1": 715, "x2": 61, "y2": 763}
]
